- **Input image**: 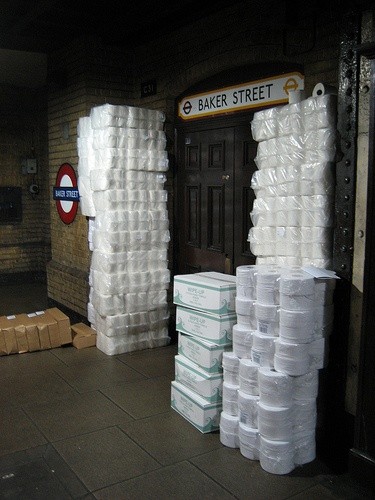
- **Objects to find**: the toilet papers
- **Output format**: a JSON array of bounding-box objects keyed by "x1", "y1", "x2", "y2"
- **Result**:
[
  {"x1": 247, "y1": 82, "x2": 334, "y2": 264},
  {"x1": 219, "y1": 262, "x2": 326, "y2": 475},
  {"x1": 76, "y1": 103, "x2": 172, "y2": 356}
]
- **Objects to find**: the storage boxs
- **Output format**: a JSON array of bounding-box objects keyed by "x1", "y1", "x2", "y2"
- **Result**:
[
  {"x1": 0, "y1": 308, "x2": 97, "y2": 354},
  {"x1": 170, "y1": 271, "x2": 236, "y2": 434}
]
